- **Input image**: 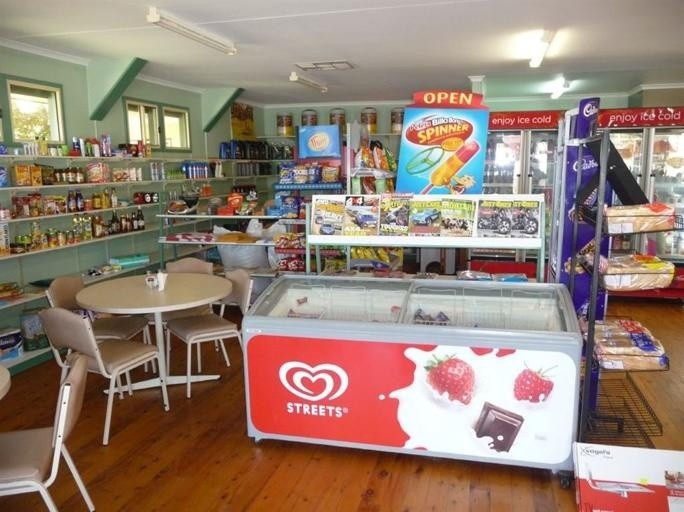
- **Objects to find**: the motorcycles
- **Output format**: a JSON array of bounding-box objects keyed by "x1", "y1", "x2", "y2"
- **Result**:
[{"x1": 480, "y1": 203, "x2": 538, "y2": 235}]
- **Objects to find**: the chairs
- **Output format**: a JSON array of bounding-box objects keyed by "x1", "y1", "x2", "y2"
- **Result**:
[
  {"x1": 0, "y1": 350, "x2": 108, "y2": 510},
  {"x1": 139, "y1": 251, "x2": 230, "y2": 382},
  {"x1": 165, "y1": 265, "x2": 260, "y2": 399},
  {"x1": 42, "y1": 273, "x2": 161, "y2": 401},
  {"x1": 32, "y1": 307, "x2": 161, "y2": 449}
]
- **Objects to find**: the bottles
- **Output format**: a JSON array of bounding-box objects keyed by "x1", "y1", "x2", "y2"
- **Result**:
[
  {"x1": 67, "y1": 187, "x2": 83, "y2": 211},
  {"x1": 237, "y1": 163, "x2": 272, "y2": 175},
  {"x1": 182, "y1": 160, "x2": 210, "y2": 180},
  {"x1": 144, "y1": 269, "x2": 168, "y2": 292},
  {"x1": 21, "y1": 132, "x2": 152, "y2": 157},
  {"x1": 73, "y1": 204, "x2": 146, "y2": 242},
  {"x1": 110, "y1": 187, "x2": 118, "y2": 208}
]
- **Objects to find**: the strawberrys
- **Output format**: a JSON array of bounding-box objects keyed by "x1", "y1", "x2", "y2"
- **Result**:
[
  {"x1": 513, "y1": 369, "x2": 554, "y2": 403},
  {"x1": 427, "y1": 357, "x2": 476, "y2": 406}
]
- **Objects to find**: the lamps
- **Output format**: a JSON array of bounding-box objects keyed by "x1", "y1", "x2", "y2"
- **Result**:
[
  {"x1": 549, "y1": 80, "x2": 569, "y2": 101},
  {"x1": 145, "y1": 6, "x2": 237, "y2": 57},
  {"x1": 288, "y1": 71, "x2": 328, "y2": 94},
  {"x1": 525, "y1": 23, "x2": 556, "y2": 69}
]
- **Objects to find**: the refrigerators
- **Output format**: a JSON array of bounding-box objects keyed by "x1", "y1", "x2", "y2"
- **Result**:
[
  {"x1": 241, "y1": 273, "x2": 583, "y2": 490},
  {"x1": 463, "y1": 112, "x2": 565, "y2": 277},
  {"x1": 576, "y1": 108, "x2": 684, "y2": 302}
]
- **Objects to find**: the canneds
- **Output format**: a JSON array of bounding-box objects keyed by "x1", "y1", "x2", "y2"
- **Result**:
[
  {"x1": 55, "y1": 167, "x2": 85, "y2": 184},
  {"x1": 14, "y1": 222, "x2": 80, "y2": 250}
]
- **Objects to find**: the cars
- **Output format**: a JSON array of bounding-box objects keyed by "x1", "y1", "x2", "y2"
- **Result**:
[
  {"x1": 314, "y1": 208, "x2": 335, "y2": 235},
  {"x1": 349, "y1": 206, "x2": 378, "y2": 230},
  {"x1": 384, "y1": 204, "x2": 408, "y2": 226},
  {"x1": 412, "y1": 208, "x2": 441, "y2": 226}
]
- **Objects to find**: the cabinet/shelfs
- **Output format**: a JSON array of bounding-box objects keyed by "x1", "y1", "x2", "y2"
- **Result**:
[
  {"x1": 346, "y1": 119, "x2": 405, "y2": 279},
  {"x1": 272, "y1": 179, "x2": 344, "y2": 278},
  {"x1": 0, "y1": 138, "x2": 296, "y2": 376},
  {"x1": 577, "y1": 131, "x2": 680, "y2": 445},
  {"x1": 154, "y1": 213, "x2": 277, "y2": 310},
  {"x1": 305, "y1": 198, "x2": 546, "y2": 284}
]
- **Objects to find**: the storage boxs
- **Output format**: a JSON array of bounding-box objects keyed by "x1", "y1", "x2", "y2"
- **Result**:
[{"x1": 572, "y1": 441, "x2": 684, "y2": 512}]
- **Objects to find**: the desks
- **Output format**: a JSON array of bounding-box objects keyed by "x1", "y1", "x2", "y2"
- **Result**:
[
  {"x1": 2, "y1": 355, "x2": 17, "y2": 403},
  {"x1": 75, "y1": 263, "x2": 223, "y2": 408}
]
